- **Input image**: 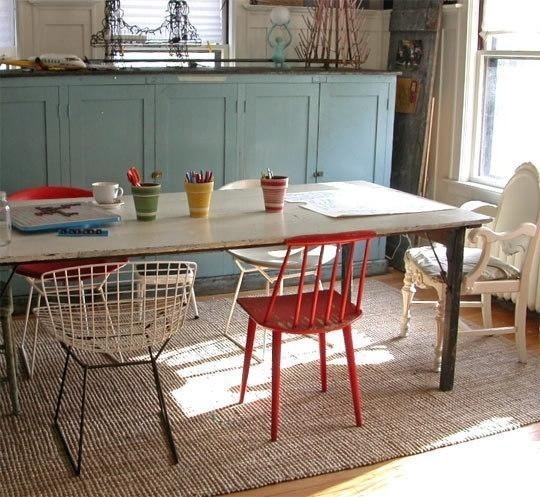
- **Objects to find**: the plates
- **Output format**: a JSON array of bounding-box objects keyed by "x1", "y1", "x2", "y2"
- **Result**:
[{"x1": 94, "y1": 198, "x2": 123, "y2": 209}]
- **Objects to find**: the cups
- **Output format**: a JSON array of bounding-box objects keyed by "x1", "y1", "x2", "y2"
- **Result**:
[{"x1": 93, "y1": 181, "x2": 124, "y2": 203}]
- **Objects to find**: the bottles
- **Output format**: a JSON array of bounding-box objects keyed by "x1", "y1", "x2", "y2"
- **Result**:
[{"x1": 0, "y1": 190, "x2": 13, "y2": 247}]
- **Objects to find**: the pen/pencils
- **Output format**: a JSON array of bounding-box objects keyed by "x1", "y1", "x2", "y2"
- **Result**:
[
  {"x1": 60, "y1": 228, "x2": 102, "y2": 234},
  {"x1": 185, "y1": 169, "x2": 212, "y2": 183},
  {"x1": 267, "y1": 168, "x2": 274, "y2": 179}
]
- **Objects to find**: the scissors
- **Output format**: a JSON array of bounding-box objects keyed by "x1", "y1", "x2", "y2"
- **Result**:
[{"x1": 127, "y1": 166, "x2": 140, "y2": 187}]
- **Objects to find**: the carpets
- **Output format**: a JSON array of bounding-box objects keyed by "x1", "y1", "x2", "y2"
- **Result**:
[{"x1": 0, "y1": 277, "x2": 540, "y2": 497}]
